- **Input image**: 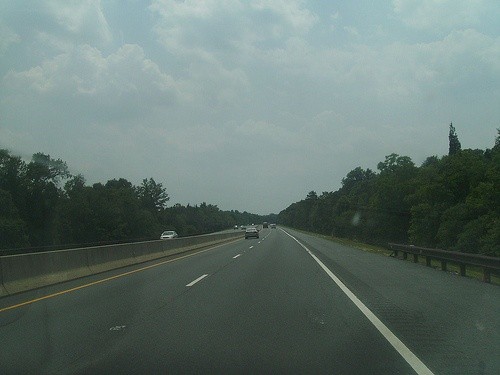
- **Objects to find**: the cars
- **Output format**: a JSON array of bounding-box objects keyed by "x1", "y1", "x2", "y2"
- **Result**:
[
  {"x1": 233, "y1": 225, "x2": 238, "y2": 230},
  {"x1": 244, "y1": 227, "x2": 259, "y2": 239},
  {"x1": 271, "y1": 223, "x2": 276, "y2": 229},
  {"x1": 262, "y1": 221, "x2": 268, "y2": 228},
  {"x1": 159, "y1": 230, "x2": 179, "y2": 240}
]
